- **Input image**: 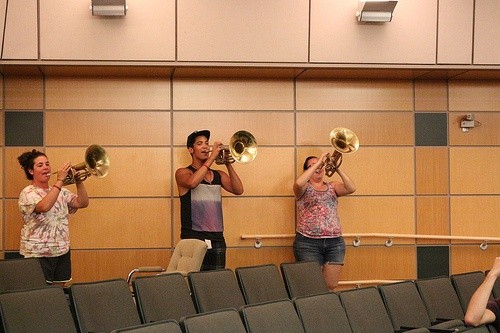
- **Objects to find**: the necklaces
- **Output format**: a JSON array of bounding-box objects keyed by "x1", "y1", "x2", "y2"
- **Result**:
[{"x1": 191, "y1": 164, "x2": 212, "y2": 185}]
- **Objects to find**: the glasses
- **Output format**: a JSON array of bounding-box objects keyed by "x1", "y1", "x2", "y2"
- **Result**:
[{"x1": 306, "y1": 164, "x2": 312, "y2": 169}]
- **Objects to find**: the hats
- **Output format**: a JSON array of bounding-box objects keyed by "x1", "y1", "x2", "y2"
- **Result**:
[{"x1": 186, "y1": 130, "x2": 211, "y2": 147}]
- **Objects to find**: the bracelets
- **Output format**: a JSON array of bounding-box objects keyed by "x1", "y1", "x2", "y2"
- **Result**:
[
  {"x1": 202, "y1": 164, "x2": 209, "y2": 169},
  {"x1": 53, "y1": 185, "x2": 61, "y2": 191}
]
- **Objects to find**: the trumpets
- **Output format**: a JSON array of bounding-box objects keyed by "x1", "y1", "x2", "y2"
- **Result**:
[
  {"x1": 202, "y1": 130, "x2": 258, "y2": 165},
  {"x1": 316, "y1": 127, "x2": 360, "y2": 179},
  {"x1": 45, "y1": 143, "x2": 112, "y2": 187}
]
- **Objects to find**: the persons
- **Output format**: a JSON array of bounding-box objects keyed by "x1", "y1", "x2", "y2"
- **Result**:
[
  {"x1": 175, "y1": 129, "x2": 245, "y2": 271},
  {"x1": 292, "y1": 152, "x2": 356, "y2": 291},
  {"x1": 17, "y1": 149, "x2": 88, "y2": 285},
  {"x1": 464, "y1": 256, "x2": 500, "y2": 333}
]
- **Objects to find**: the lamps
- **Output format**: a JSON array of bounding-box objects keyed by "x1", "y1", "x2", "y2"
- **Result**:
[
  {"x1": 90, "y1": 0, "x2": 128, "y2": 18},
  {"x1": 357, "y1": 1, "x2": 397, "y2": 23}
]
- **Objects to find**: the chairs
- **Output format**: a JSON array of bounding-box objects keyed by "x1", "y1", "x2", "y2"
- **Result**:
[
  {"x1": 235, "y1": 263, "x2": 291, "y2": 304},
  {"x1": 280, "y1": 260, "x2": 331, "y2": 298},
  {"x1": 0, "y1": 257, "x2": 48, "y2": 294},
  {"x1": 415, "y1": 277, "x2": 490, "y2": 333},
  {"x1": 111, "y1": 307, "x2": 247, "y2": 333},
  {"x1": 127, "y1": 239, "x2": 208, "y2": 298},
  {"x1": 450, "y1": 268, "x2": 500, "y2": 333},
  {"x1": 131, "y1": 272, "x2": 198, "y2": 324},
  {"x1": 378, "y1": 280, "x2": 464, "y2": 333},
  {"x1": 292, "y1": 292, "x2": 353, "y2": 333},
  {"x1": 0, "y1": 285, "x2": 78, "y2": 333},
  {"x1": 239, "y1": 297, "x2": 305, "y2": 333},
  {"x1": 188, "y1": 268, "x2": 246, "y2": 314},
  {"x1": 69, "y1": 277, "x2": 142, "y2": 333},
  {"x1": 338, "y1": 286, "x2": 430, "y2": 333}
]
